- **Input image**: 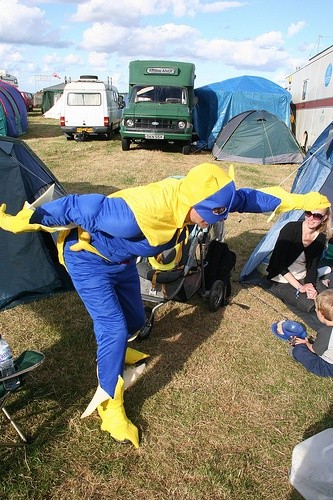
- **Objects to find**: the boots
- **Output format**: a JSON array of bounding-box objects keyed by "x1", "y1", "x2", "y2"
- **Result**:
[
  {"x1": 123, "y1": 329, "x2": 149, "y2": 364},
  {"x1": 80, "y1": 365, "x2": 140, "y2": 450}
]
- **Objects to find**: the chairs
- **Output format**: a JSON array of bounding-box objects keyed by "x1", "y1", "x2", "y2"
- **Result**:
[{"x1": 0, "y1": 350, "x2": 46, "y2": 445}]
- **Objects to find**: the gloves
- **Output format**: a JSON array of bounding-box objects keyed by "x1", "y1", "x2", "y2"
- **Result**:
[
  {"x1": 255, "y1": 186, "x2": 330, "y2": 223},
  {"x1": 0, "y1": 201, "x2": 57, "y2": 234}
]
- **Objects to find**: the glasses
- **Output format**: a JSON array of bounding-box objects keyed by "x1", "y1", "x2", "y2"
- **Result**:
[{"x1": 305, "y1": 211, "x2": 325, "y2": 220}]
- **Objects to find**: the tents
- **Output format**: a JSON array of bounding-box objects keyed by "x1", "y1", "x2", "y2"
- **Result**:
[
  {"x1": 0, "y1": 80, "x2": 29, "y2": 136},
  {"x1": 211, "y1": 110, "x2": 306, "y2": 164},
  {"x1": 240, "y1": 120, "x2": 333, "y2": 281},
  {"x1": 0, "y1": 136, "x2": 75, "y2": 311}
]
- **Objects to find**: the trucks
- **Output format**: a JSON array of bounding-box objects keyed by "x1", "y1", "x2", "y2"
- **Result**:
[{"x1": 119, "y1": 59, "x2": 199, "y2": 155}]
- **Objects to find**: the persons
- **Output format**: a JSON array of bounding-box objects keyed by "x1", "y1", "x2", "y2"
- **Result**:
[
  {"x1": 289, "y1": 289, "x2": 333, "y2": 377},
  {"x1": 0, "y1": 164, "x2": 331, "y2": 447},
  {"x1": 266, "y1": 205, "x2": 333, "y2": 313}
]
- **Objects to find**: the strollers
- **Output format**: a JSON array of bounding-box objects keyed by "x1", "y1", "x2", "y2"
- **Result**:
[{"x1": 135, "y1": 175, "x2": 227, "y2": 341}]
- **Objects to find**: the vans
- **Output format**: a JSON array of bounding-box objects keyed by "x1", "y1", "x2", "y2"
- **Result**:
[
  {"x1": 59, "y1": 74, "x2": 123, "y2": 140},
  {"x1": 19, "y1": 91, "x2": 35, "y2": 112}
]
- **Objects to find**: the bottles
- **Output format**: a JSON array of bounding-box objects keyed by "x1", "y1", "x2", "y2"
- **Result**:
[{"x1": 0, "y1": 334, "x2": 20, "y2": 390}]
